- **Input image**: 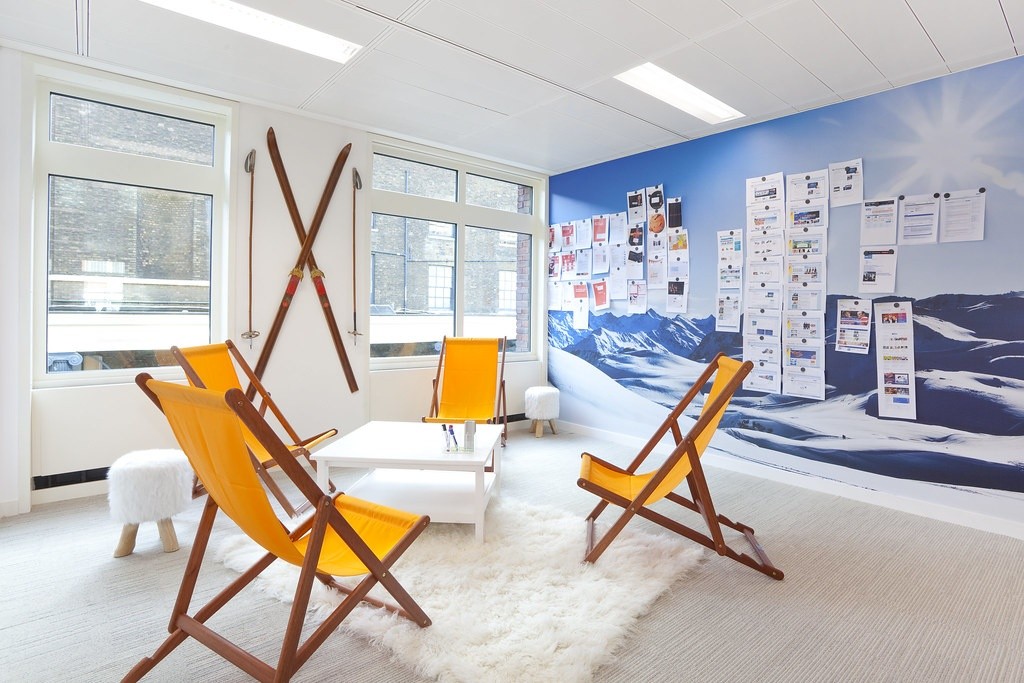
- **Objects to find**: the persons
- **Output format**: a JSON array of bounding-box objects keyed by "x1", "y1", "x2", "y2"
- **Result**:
[
  {"x1": 881, "y1": 314, "x2": 898, "y2": 324},
  {"x1": 857, "y1": 311, "x2": 869, "y2": 325},
  {"x1": 842, "y1": 311, "x2": 855, "y2": 326}
]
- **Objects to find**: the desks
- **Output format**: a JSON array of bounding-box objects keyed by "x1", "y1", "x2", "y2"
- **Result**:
[{"x1": 309, "y1": 420, "x2": 505, "y2": 546}]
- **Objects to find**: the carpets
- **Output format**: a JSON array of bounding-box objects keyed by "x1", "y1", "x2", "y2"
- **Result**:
[{"x1": 215, "y1": 495, "x2": 706, "y2": 683}]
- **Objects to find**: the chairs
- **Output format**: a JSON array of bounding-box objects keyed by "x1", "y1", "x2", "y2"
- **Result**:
[
  {"x1": 575, "y1": 352, "x2": 784, "y2": 581},
  {"x1": 420, "y1": 334, "x2": 507, "y2": 473},
  {"x1": 171, "y1": 339, "x2": 337, "y2": 520},
  {"x1": 119, "y1": 371, "x2": 434, "y2": 683}
]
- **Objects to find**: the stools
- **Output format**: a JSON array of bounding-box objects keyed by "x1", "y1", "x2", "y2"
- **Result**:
[
  {"x1": 105, "y1": 448, "x2": 195, "y2": 558},
  {"x1": 523, "y1": 385, "x2": 561, "y2": 439}
]
